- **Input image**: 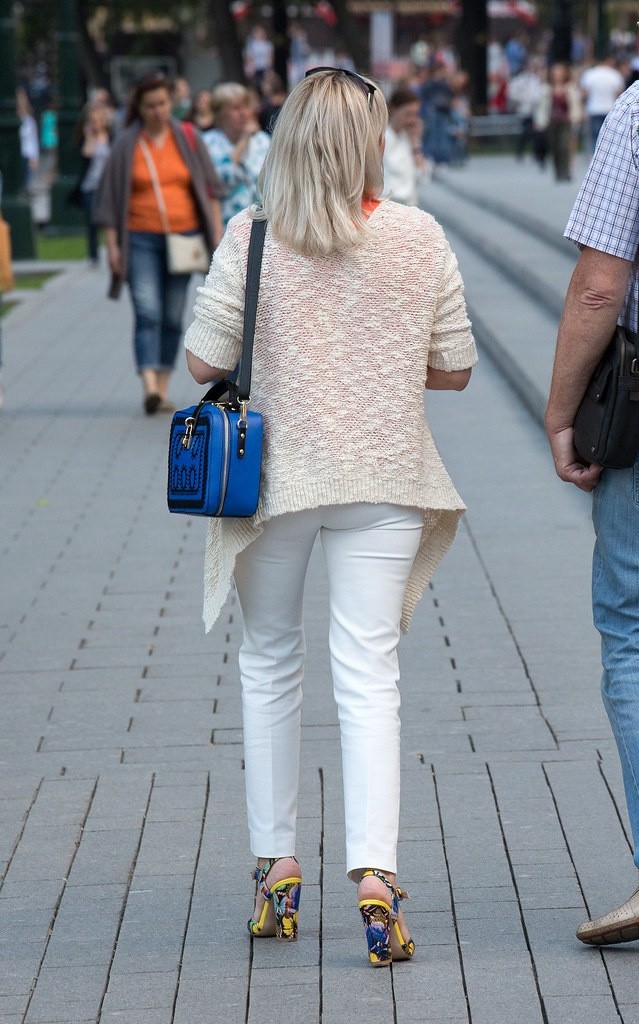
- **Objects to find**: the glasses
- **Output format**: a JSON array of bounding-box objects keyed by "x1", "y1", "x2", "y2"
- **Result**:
[{"x1": 305, "y1": 67, "x2": 376, "y2": 112}]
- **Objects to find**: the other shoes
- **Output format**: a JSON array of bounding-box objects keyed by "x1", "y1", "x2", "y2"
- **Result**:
[
  {"x1": 576, "y1": 886, "x2": 639, "y2": 945},
  {"x1": 146, "y1": 394, "x2": 174, "y2": 415}
]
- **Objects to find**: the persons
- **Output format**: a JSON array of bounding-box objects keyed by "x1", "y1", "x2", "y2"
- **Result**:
[
  {"x1": 0, "y1": 25, "x2": 286, "y2": 413},
  {"x1": 542, "y1": 82, "x2": 639, "y2": 946},
  {"x1": 289, "y1": 26, "x2": 639, "y2": 208},
  {"x1": 181, "y1": 65, "x2": 482, "y2": 969}
]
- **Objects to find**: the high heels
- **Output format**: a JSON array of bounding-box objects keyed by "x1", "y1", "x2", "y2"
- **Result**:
[
  {"x1": 247, "y1": 856, "x2": 302, "y2": 942},
  {"x1": 358, "y1": 870, "x2": 415, "y2": 968}
]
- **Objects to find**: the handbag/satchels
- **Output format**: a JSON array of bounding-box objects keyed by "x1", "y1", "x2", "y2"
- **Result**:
[
  {"x1": 574, "y1": 325, "x2": 639, "y2": 470},
  {"x1": 166, "y1": 232, "x2": 210, "y2": 273},
  {"x1": 167, "y1": 379, "x2": 263, "y2": 518}
]
